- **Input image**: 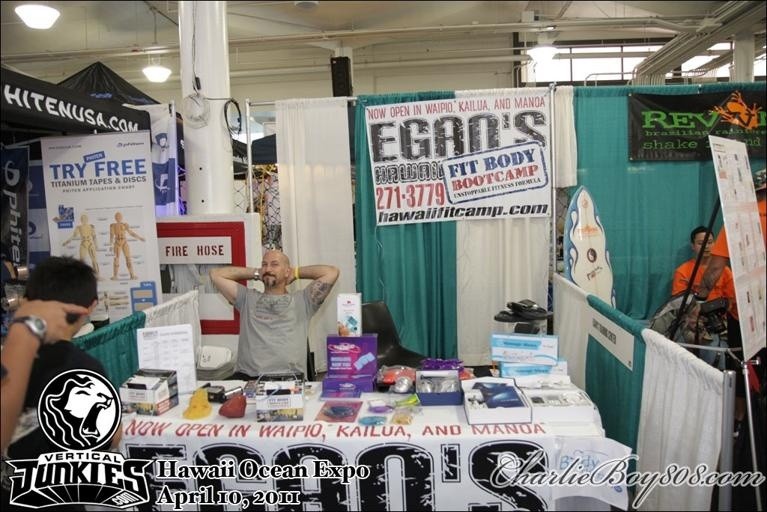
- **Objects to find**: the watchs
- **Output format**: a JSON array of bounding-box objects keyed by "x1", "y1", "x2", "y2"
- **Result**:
[{"x1": 9, "y1": 314, "x2": 47, "y2": 348}]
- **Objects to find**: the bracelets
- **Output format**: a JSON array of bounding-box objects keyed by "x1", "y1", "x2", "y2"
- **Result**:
[
  {"x1": 254, "y1": 267, "x2": 262, "y2": 281},
  {"x1": 294, "y1": 266, "x2": 300, "y2": 281},
  {"x1": 694, "y1": 292, "x2": 706, "y2": 306}
]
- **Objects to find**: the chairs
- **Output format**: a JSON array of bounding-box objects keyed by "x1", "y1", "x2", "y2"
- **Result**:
[{"x1": 358, "y1": 300, "x2": 425, "y2": 369}]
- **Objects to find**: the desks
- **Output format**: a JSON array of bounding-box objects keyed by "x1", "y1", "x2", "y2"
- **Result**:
[{"x1": 105, "y1": 377, "x2": 607, "y2": 512}]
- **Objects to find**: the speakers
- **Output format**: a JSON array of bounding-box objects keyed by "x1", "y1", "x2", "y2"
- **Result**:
[{"x1": 331, "y1": 57, "x2": 352, "y2": 96}]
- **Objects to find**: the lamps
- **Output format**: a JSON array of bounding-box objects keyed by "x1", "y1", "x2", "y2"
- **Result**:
[
  {"x1": 141, "y1": 54, "x2": 173, "y2": 84},
  {"x1": 13, "y1": 0, "x2": 61, "y2": 30}
]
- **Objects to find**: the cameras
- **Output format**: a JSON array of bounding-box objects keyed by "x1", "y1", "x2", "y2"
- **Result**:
[{"x1": 700, "y1": 297, "x2": 729, "y2": 334}]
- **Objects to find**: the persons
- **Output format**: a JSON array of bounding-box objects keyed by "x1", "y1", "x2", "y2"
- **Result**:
[
  {"x1": 61, "y1": 214, "x2": 101, "y2": 275},
  {"x1": 691, "y1": 193, "x2": 765, "y2": 328},
  {"x1": 670, "y1": 224, "x2": 742, "y2": 322},
  {"x1": 1, "y1": 256, "x2": 122, "y2": 512},
  {"x1": 1, "y1": 299, "x2": 89, "y2": 459},
  {"x1": 208, "y1": 249, "x2": 340, "y2": 382},
  {"x1": 109, "y1": 212, "x2": 147, "y2": 281}
]
